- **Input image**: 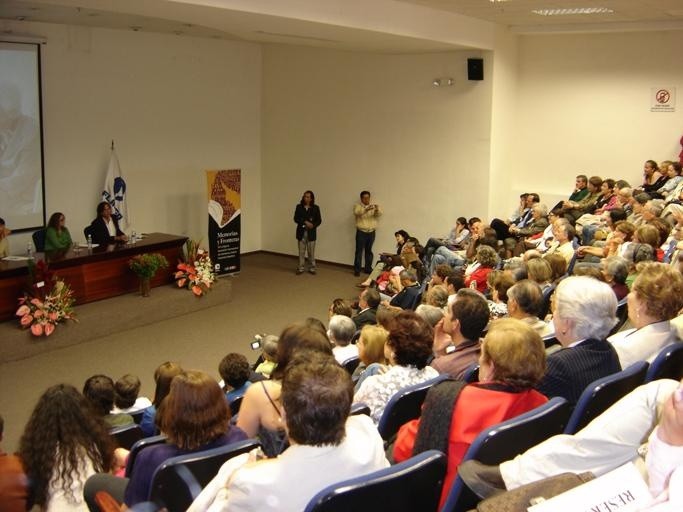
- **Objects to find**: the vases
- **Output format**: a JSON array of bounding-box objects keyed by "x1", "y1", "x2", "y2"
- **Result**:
[{"x1": 140, "y1": 277, "x2": 152, "y2": 297}]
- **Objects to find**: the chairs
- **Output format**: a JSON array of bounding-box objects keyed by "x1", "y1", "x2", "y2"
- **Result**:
[
  {"x1": 108, "y1": 396, "x2": 262, "y2": 512},
  {"x1": 377, "y1": 374, "x2": 458, "y2": 439},
  {"x1": 539, "y1": 240, "x2": 676, "y2": 349},
  {"x1": 351, "y1": 330, "x2": 361, "y2": 343},
  {"x1": 278, "y1": 403, "x2": 369, "y2": 455},
  {"x1": 411, "y1": 291, "x2": 422, "y2": 312},
  {"x1": 441, "y1": 396, "x2": 569, "y2": 512},
  {"x1": 463, "y1": 362, "x2": 479, "y2": 384},
  {"x1": 562, "y1": 361, "x2": 650, "y2": 435},
  {"x1": 33, "y1": 230, "x2": 46, "y2": 251},
  {"x1": 303, "y1": 449, "x2": 447, "y2": 512},
  {"x1": 84, "y1": 225, "x2": 97, "y2": 244},
  {"x1": 342, "y1": 356, "x2": 360, "y2": 374},
  {"x1": 645, "y1": 342, "x2": 683, "y2": 384}
]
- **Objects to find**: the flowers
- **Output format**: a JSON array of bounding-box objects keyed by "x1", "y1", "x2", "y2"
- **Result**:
[
  {"x1": 17, "y1": 259, "x2": 79, "y2": 336},
  {"x1": 129, "y1": 252, "x2": 169, "y2": 279},
  {"x1": 174, "y1": 237, "x2": 219, "y2": 297}
]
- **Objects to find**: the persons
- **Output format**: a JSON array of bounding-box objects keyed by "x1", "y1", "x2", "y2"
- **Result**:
[
  {"x1": 0, "y1": 162, "x2": 683, "y2": 511},
  {"x1": 0, "y1": 81, "x2": 40, "y2": 212}
]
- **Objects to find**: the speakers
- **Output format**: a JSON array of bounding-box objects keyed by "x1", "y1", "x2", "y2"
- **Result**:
[{"x1": 466, "y1": 56, "x2": 484, "y2": 81}]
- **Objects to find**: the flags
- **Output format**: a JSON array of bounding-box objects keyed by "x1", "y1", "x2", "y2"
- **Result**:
[{"x1": 103, "y1": 147, "x2": 128, "y2": 234}]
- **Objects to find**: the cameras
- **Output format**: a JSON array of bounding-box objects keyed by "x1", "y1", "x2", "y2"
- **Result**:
[
  {"x1": 368, "y1": 204, "x2": 376, "y2": 209},
  {"x1": 251, "y1": 334, "x2": 266, "y2": 352}
]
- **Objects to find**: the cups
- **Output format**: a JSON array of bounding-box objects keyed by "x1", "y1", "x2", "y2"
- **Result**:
[{"x1": 73, "y1": 240, "x2": 79, "y2": 252}]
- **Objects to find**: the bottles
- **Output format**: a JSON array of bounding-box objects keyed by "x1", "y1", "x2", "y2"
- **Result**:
[
  {"x1": 131, "y1": 231, "x2": 136, "y2": 244},
  {"x1": 28, "y1": 242, "x2": 32, "y2": 257},
  {"x1": 88, "y1": 234, "x2": 92, "y2": 250}
]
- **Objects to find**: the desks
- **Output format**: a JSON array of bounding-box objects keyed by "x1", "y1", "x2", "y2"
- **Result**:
[{"x1": 1, "y1": 232, "x2": 189, "y2": 323}]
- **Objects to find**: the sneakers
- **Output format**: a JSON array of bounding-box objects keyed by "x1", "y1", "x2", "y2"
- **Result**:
[
  {"x1": 296, "y1": 269, "x2": 304, "y2": 275},
  {"x1": 308, "y1": 269, "x2": 316, "y2": 275}
]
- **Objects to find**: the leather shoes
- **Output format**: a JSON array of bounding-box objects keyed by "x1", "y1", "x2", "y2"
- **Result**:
[
  {"x1": 458, "y1": 460, "x2": 507, "y2": 501},
  {"x1": 351, "y1": 300, "x2": 358, "y2": 309},
  {"x1": 354, "y1": 271, "x2": 360, "y2": 277},
  {"x1": 365, "y1": 271, "x2": 371, "y2": 275},
  {"x1": 355, "y1": 283, "x2": 370, "y2": 288}
]
